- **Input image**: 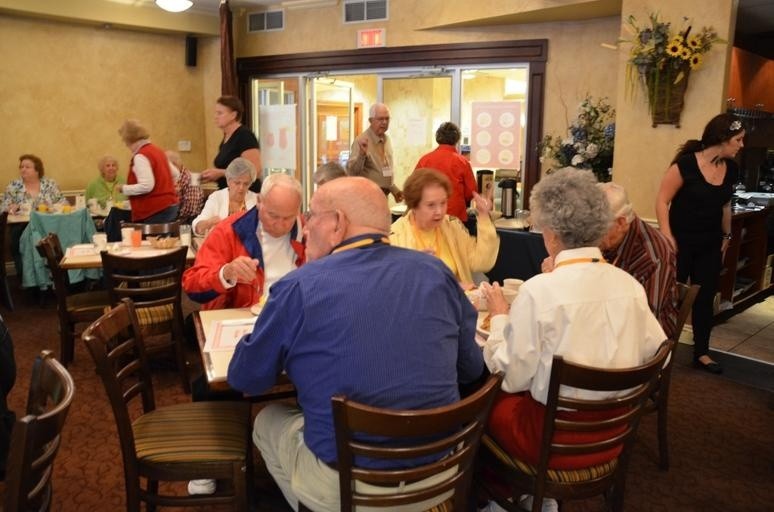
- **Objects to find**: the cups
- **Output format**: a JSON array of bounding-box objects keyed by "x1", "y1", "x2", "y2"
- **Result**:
[
  {"x1": 492, "y1": 276, "x2": 523, "y2": 306},
  {"x1": 109, "y1": 190, "x2": 128, "y2": 210},
  {"x1": 178, "y1": 224, "x2": 192, "y2": 248},
  {"x1": 91, "y1": 233, "x2": 106, "y2": 252},
  {"x1": 190, "y1": 173, "x2": 202, "y2": 187},
  {"x1": 121, "y1": 227, "x2": 143, "y2": 247},
  {"x1": 516, "y1": 208, "x2": 530, "y2": 226}
]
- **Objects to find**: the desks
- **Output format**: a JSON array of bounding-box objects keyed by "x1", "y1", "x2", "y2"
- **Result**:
[{"x1": 719, "y1": 201, "x2": 774, "y2": 304}]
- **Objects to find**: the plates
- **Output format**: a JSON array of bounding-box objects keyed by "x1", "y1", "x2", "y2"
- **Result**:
[
  {"x1": 476, "y1": 319, "x2": 489, "y2": 339},
  {"x1": 250, "y1": 303, "x2": 260, "y2": 316}
]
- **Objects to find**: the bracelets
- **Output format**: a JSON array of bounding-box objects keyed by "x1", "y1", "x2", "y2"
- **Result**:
[{"x1": 723, "y1": 232, "x2": 735, "y2": 241}]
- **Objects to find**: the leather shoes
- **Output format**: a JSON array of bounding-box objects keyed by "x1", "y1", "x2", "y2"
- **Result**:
[{"x1": 693, "y1": 353, "x2": 723, "y2": 375}]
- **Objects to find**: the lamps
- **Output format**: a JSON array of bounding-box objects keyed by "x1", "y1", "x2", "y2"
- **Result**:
[
  {"x1": 156, "y1": 0, "x2": 194, "y2": 13},
  {"x1": 326, "y1": 113, "x2": 338, "y2": 142}
]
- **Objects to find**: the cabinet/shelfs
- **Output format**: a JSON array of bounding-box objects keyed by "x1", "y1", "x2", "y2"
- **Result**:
[{"x1": 731, "y1": 107, "x2": 774, "y2": 191}]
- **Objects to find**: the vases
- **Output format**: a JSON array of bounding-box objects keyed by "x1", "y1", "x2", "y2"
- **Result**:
[{"x1": 642, "y1": 69, "x2": 688, "y2": 129}]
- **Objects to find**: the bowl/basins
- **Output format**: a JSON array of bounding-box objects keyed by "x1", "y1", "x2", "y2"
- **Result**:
[{"x1": 145, "y1": 236, "x2": 178, "y2": 248}]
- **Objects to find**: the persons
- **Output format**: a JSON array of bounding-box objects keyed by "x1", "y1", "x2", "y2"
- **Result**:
[
  {"x1": 597, "y1": 182, "x2": 678, "y2": 340},
  {"x1": 345, "y1": 104, "x2": 405, "y2": 205},
  {"x1": 192, "y1": 157, "x2": 258, "y2": 240},
  {"x1": 181, "y1": 172, "x2": 309, "y2": 496},
  {"x1": 482, "y1": 167, "x2": 667, "y2": 512},
  {"x1": 117, "y1": 120, "x2": 180, "y2": 224},
  {"x1": 84, "y1": 156, "x2": 129, "y2": 209},
  {"x1": 227, "y1": 176, "x2": 484, "y2": 512},
  {"x1": 416, "y1": 122, "x2": 479, "y2": 223},
  {"x1": 311, "y1": 162, "x2": 345, "y2": 185},
  {"x1": 1, "y1": 151, "x2": 72, "y2": 294},
  {"x1": 164, "y1": 150, "x2": 206, "y2": 222},
  {"x1": 198, "y1": 94, "x2": 262, "y2": 193},
  {"x1": 654, "y1": 111, "x2": 746, "y2": 375},
  {"x1": 383, "y1": 166, "x2": 500, "y2": 291}
]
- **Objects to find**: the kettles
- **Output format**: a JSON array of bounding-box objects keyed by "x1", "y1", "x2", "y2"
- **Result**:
[{"x1": 498, "y1": 179, "x2": 518, "y2": 219}]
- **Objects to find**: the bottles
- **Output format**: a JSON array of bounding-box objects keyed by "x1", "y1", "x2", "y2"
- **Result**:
[{"x1": 36, "y1": 189, "x2": 72, "y2": 214}]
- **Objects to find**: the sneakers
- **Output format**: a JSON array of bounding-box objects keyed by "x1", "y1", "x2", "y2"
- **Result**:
[{"x1": 186, "y1": 480, "x2": 218, "y2": 495}]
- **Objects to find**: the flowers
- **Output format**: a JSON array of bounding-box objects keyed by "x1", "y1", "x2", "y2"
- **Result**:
[
  {"x1": 534, "y1": 90, "x2": 615, "y2": 183},
  {"x1": 599, "y1": 7, "x2": 729, "y2": 119}
]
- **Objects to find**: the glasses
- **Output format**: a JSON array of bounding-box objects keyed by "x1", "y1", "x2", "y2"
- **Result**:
[
  {"x1": 378, "y1": 117, "x2": 390, "y2": 120},
  {"x1": 306, "y1": 209, "x2": 336, "y2": 222}
]
